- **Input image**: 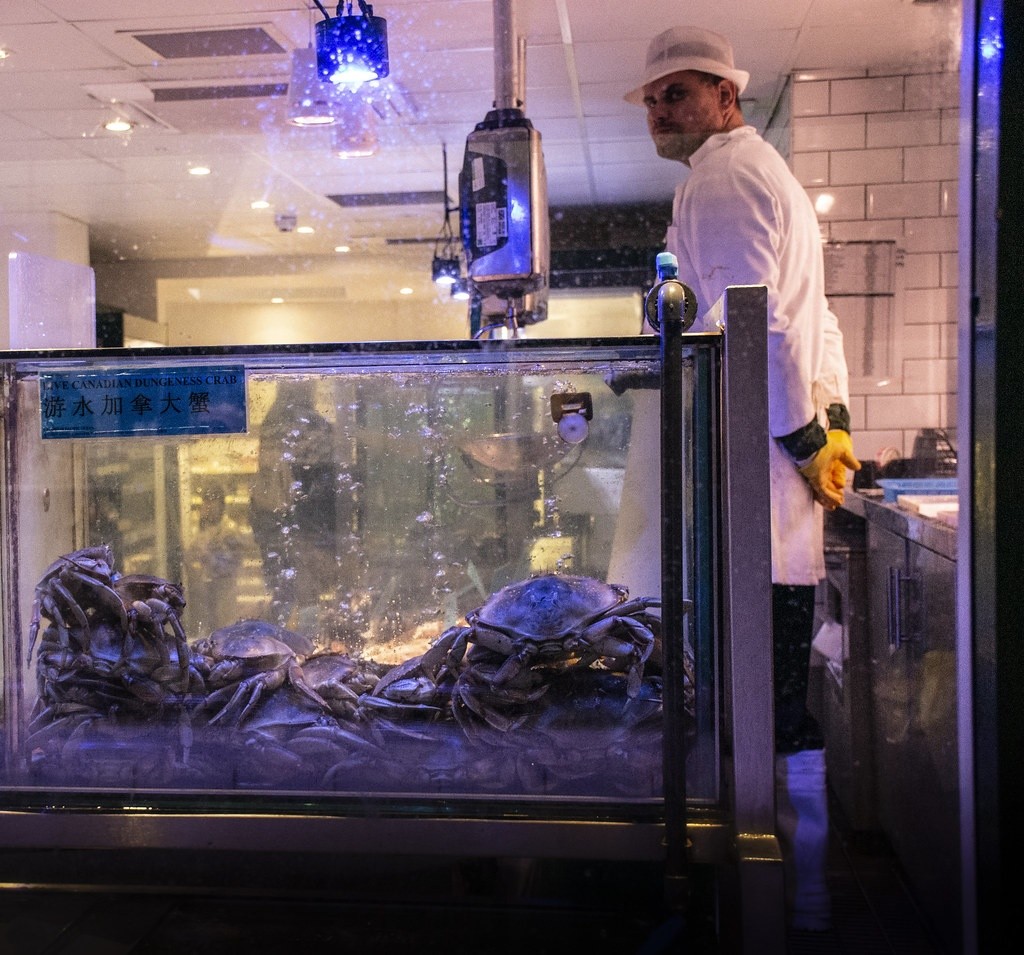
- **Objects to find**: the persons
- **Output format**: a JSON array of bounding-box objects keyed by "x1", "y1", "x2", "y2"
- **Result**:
[
  {"x1": 607, "y1": 28, "x2": 861, "y2": 935},
  {"x1": 253, "y1": 379, "x2": 336, "y2": 633},
  {"x1": 184, "y1": 491, "x2": 244, "y2": 634}
]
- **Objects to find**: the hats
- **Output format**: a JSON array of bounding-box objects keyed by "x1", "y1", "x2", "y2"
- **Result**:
[{"x1": 623, "y1": 25, "x2": 750, "y2": 107}]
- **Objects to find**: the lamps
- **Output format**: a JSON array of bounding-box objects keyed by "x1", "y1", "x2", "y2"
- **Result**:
[{"x1": 285, "y1": 1, "x2": 390, "y2": 125}]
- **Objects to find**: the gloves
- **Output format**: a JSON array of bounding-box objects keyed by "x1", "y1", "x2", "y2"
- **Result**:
[
  {"x1": 813, "y1": 429, "x2": 855, "y2": 510},
  {"x1": 800, "y1": 444, "x2": 861, "y2": 505}
]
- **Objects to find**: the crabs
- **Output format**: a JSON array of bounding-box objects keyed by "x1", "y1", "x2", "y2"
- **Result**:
[{"x1": 14, "y1": 544, "x2": 699, "y2": 796}]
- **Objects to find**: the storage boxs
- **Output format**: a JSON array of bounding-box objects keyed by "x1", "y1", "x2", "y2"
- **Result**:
[{"x1": 0, "y1": 287, "x2": 790, "y2": 864}]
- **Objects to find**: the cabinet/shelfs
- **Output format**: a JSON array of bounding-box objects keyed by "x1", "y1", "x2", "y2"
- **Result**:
[{"x1": 806, "y1": 504, "x2": 962, "y2": 952}]
